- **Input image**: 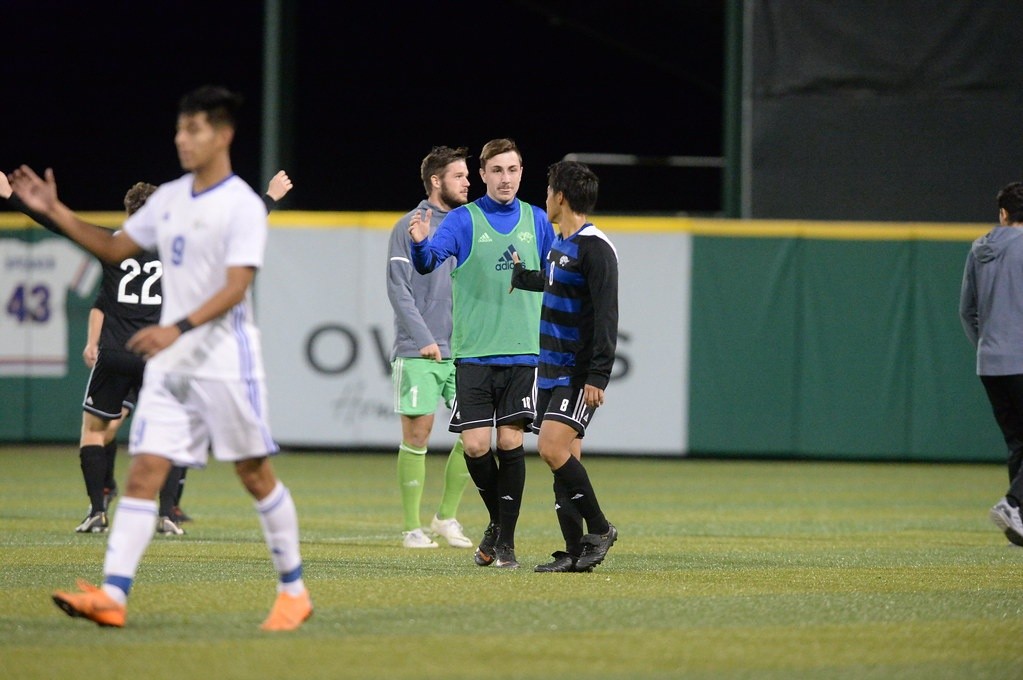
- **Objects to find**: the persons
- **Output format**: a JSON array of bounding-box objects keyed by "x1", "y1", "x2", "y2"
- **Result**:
[
  {"x1": 385, "y1": 145, "x2": 474, "y2": 549},
  {"x1": 409, "y1": 138, "x2": 556, "y2": 567},
  {"x1": 958, "y1": 183, "x2": 1023, "y2": 547},
  {"x1": 512, "y1": 160, "x2": 619, "y2": 571},
  {"x1": 0, "y1": 169, "x2": 294, "y2": 534},
  {"x1": 6, "y1": 86, "x2": 315, "y2": 631}
]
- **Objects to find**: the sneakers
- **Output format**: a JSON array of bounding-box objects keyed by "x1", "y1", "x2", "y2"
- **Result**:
[
  {"x1": 51, "y1": 579, "x2": 127, "y2": 629},
  {"x1": 75, "y1": 510, "x2": 110, "y2": 534},
  {"x1": 989, "y1": 498, "x2": 1023, "y2": 548},
  {"x1": 474, "y1": 518, "x2": 500, "y2": 566},
  {"x1": 430, "y1": 512, "x2": 475, "y2": 549},
  {"x1": 259, "y1": 583, "x2": 314, "y2": 631},
  {"x1": 156, "y1": 515, "x2": 186, "y2": 537},
  {"x1": 86, "y1": 480, "x2": 118, "y2": 515},
  {"x1": 494, "y1": 540, "x2": 520, "y2": 570},
  {"x1": 157, "y1": 506, "x2": 194, "y2": 523},
  {"x1": 575, "y1": 520, "x2": 619, "y2": 575},
  {"x1": 401, "y1": 528, "x2": 439, "y2": 549},
  {"x1": 533, "y1": 550, "x2": 593, "y2": 573}
]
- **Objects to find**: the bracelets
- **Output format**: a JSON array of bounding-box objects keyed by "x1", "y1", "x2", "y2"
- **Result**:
[{"x1": 176, "y1": 318, "x2": 194, "y2": 332}]
